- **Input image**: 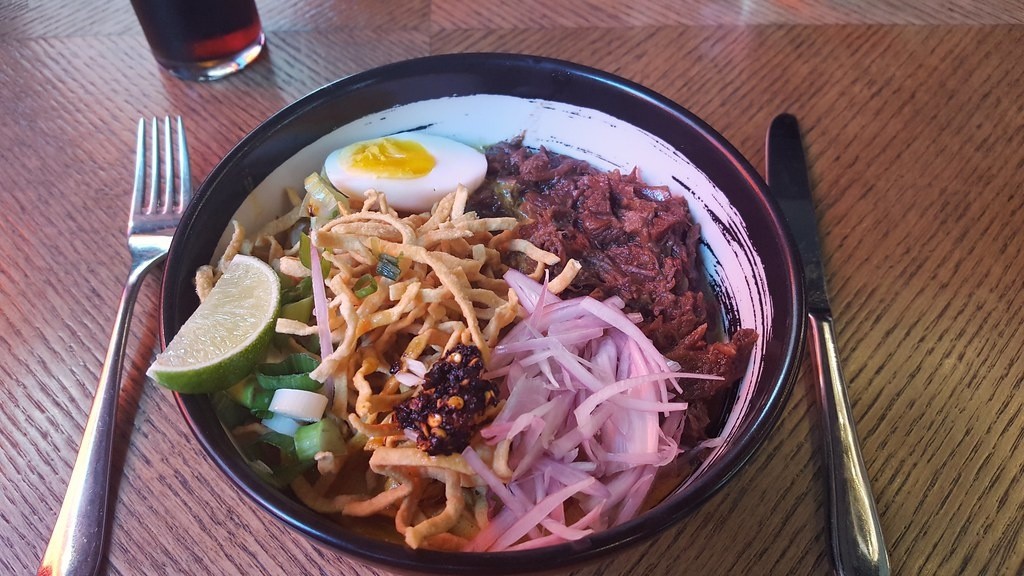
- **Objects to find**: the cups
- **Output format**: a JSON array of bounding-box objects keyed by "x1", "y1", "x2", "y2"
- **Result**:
[{"x1": 130, "y1": 0, "x2": 265, "y2": 82}]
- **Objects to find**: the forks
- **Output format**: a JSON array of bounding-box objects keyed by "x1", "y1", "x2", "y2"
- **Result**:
[{"x1": 37, "y1": 116, "x2": 192, "y2": 576}]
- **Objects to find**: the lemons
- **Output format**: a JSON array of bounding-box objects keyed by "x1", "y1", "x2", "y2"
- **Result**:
[{"x1": 144, "y1": 253, "x2": 282, "y2": 393}]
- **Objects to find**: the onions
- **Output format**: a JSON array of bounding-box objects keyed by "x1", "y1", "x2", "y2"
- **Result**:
[{"x1": 393, "y1": 268, "x2": 727, "y2": 553}]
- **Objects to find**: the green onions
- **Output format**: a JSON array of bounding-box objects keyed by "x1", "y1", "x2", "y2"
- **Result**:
[{"x1": 212, "y1": 230, "x2": 399, "y2": 488}]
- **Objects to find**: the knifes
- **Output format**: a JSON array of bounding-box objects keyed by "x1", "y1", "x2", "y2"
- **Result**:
[{"x1": 764, "y1": 113, "x2": 889, "y2": 576}]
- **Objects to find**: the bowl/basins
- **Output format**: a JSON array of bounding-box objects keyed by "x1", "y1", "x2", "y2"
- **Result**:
[{"x1": 157, "y1": 50, "x2": 807, "y2": 573}]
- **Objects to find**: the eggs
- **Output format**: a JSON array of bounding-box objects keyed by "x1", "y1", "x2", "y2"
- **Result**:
[{"x1": 325, "y1": 133, "x2": 488, "y2": 213}]
